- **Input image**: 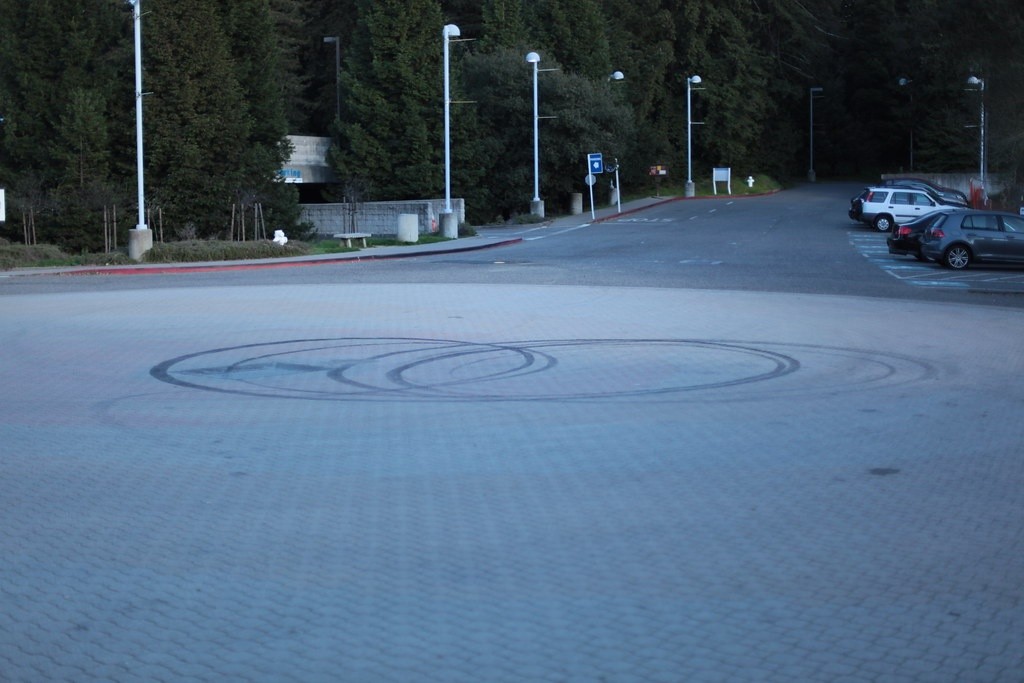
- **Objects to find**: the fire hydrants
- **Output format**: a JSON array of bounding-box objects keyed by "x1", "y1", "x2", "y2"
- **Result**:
[
  {"x1": 747, "y1": 176, "x2": 755, "y2": 187},
  {"x1": 273, "y1": 230, "x2": 288, "y2": 245}
]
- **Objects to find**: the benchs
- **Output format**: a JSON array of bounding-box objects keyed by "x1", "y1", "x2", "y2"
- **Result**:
[{"x1": 334, "y1": 233, "x2": 372, "y2": 248}]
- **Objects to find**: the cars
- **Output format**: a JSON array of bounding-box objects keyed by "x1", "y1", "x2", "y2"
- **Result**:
[
  {"x1": 887, "y1": 208, "x2": 1019, "y2": 263},
  {"x1": 878, "y1": 176, "x2": 968, "y2": 202},
  {"x1": 885, "y1": 184, "x2": 967, "y2": 206},
  {"x1": 919, "y1": 210, "x2": 1024, "y2": 270}
]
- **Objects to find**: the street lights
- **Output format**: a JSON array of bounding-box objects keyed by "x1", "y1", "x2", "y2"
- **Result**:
[
  {"x1": 808, "y1": 87, "x2": 823, "y2": 182},
  {"x1": 443, "y1": 23, "x2": 461, "y2": 212},
  {"x1": 526, "y1": 52, "x2": 545, "y2": 220},
  {"x1": 900, "y1": 77, "x2": 915, "y2": 172},
  {"x1": 968, "y1": 76, "x2": 985, "y2": 191},
  {"x1": 683, "y1": 76, "x2": 702, "y2": 197}
]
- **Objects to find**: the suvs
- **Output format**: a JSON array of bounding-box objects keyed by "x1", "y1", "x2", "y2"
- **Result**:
[{"x1": 847, "y1": 187, "x2": 961, "y2": 233}]
